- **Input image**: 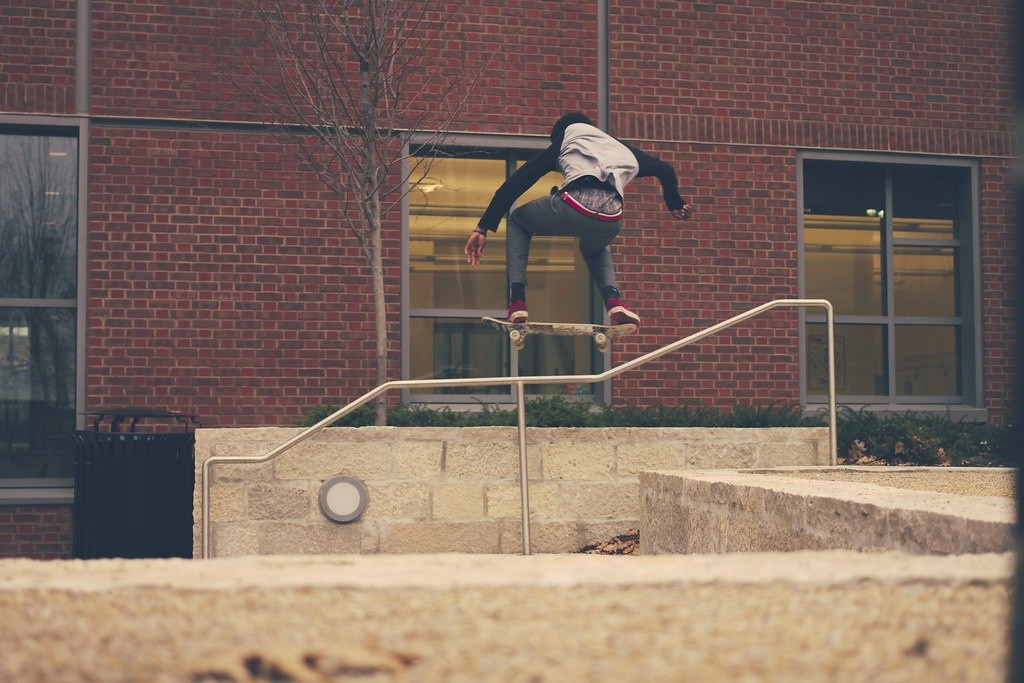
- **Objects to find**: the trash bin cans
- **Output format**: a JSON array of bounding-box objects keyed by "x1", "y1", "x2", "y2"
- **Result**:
[{"x1": 65, "y1": 406, "x2": 198, "y2": 560}]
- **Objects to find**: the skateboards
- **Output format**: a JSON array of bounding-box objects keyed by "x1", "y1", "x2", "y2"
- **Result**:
[{"x1": 481, "y1": 315, "x2": 637, "y2": 353}]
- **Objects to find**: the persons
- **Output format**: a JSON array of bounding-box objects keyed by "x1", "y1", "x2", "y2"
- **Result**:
[{"x1": 463, "y1": 112, "x2": 691, "y2": 326}]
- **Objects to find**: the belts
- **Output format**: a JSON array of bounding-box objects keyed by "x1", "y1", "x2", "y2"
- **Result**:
[{"x1": 562, "y1": 192, "x2": 623, "y2": 221}]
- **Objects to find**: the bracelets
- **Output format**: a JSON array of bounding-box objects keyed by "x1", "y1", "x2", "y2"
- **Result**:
[{"x1": 471, "y1": 229, "x2": 486, "y2": 238}]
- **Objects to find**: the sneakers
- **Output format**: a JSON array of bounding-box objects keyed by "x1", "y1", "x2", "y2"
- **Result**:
[
  {"x1": 508, "y1": 300, "x2": 528, "y2": 323},
  {"x1": 606, "y1": 299, "x2": 640, "y2": 327}
]
